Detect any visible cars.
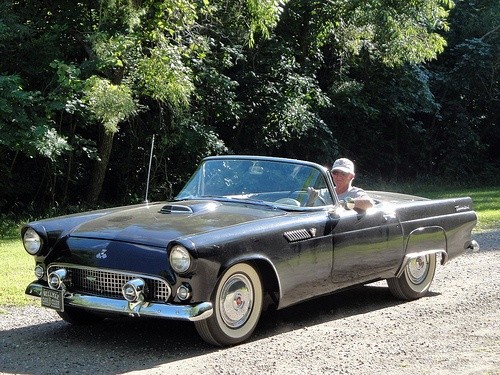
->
[22,156,480,348]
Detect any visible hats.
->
[330,157,354,174]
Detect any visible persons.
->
[302,158,376,209]
